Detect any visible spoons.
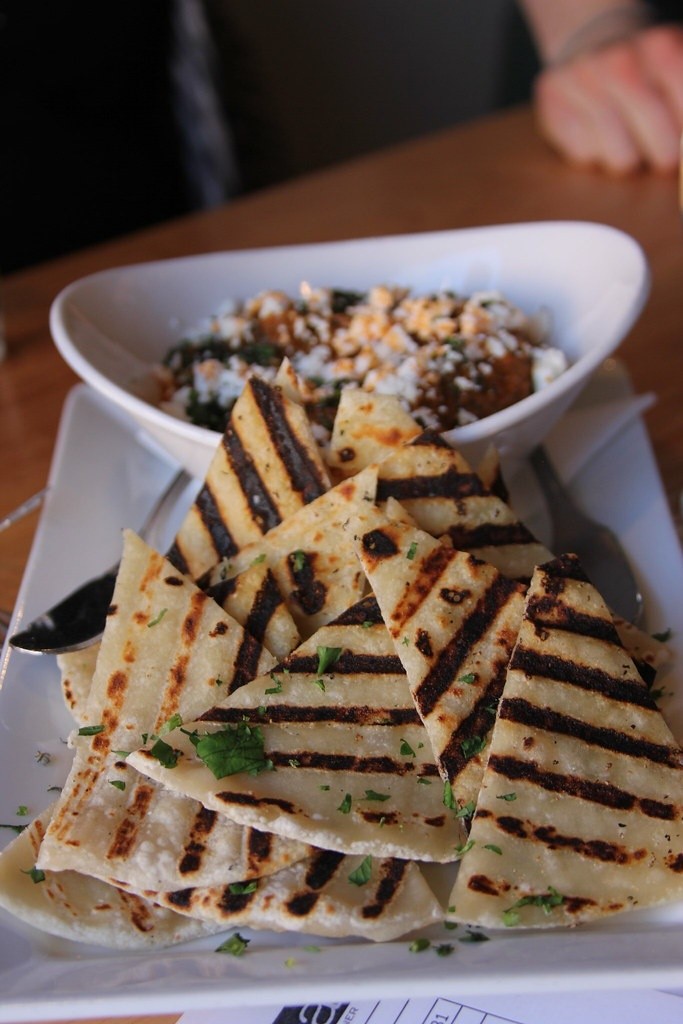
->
[5,468,196,658]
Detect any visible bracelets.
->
[550,8,651,66]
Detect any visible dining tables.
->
[0,97,683,1024]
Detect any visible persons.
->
[1,0,683,276]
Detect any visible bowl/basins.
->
[49,221,653,486]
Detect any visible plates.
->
[0,359,683,1022]
